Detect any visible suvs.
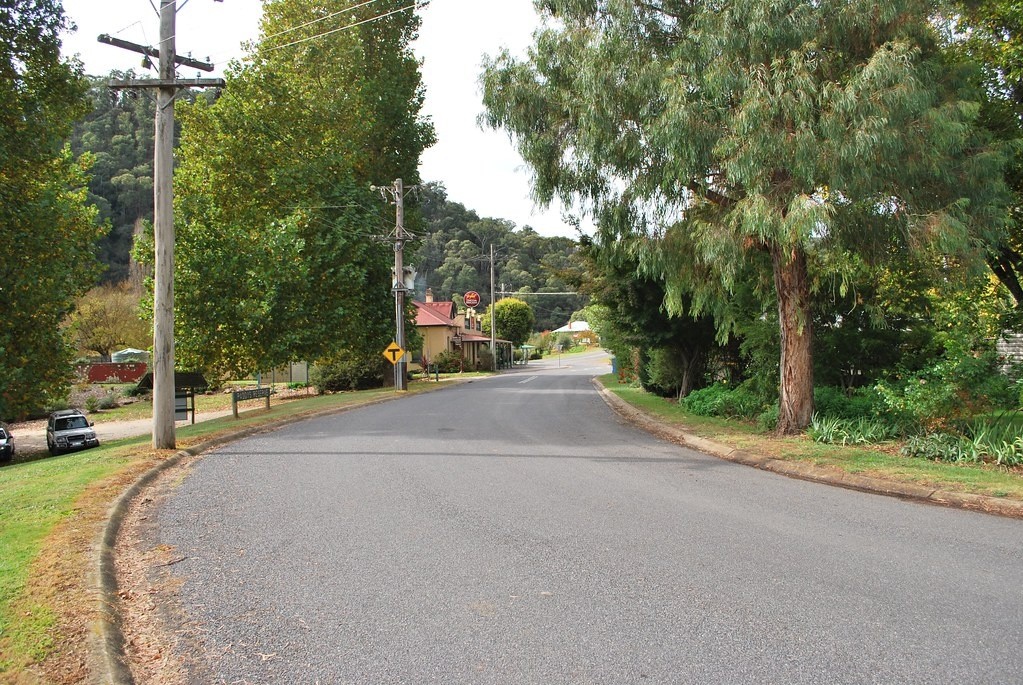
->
[47,408,99,455]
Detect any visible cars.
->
[0,427,15,463]
